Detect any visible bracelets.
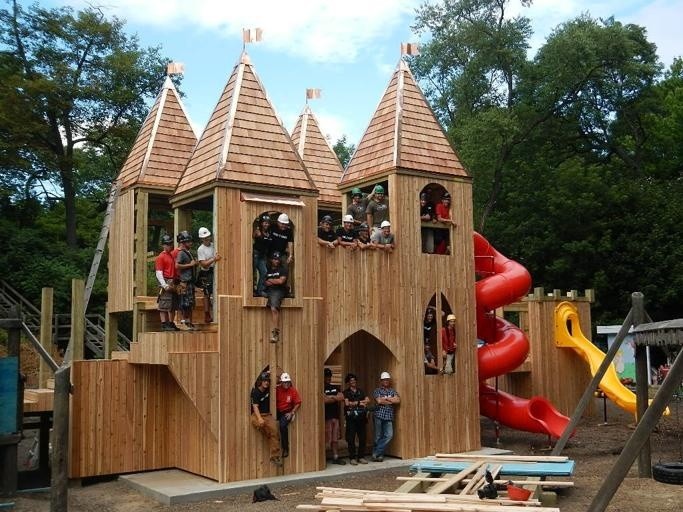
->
[211,256,216,262]
[385,396,388,401]
[356,400,360,406]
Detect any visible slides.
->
[474,230,576,439]
[554,302,670,418]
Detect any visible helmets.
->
[259,372,292,382]
[375,185,384,193]
[440,192,450,199]
[447,314,456,321]
[345,374,355,383]
[261,213,290,224]
[381,372,391,379]
[273,252,280,259]
[420,193,428,200]
[351,188,361,197]
[162,227,211,243]
[324,368,332,377]
[322,215,390,231]
[426,308,435,314]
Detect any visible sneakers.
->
[333,453,382,465]
[161,321,199,331]
[271,449,288,466]
[271,329,279,342]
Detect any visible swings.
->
[653,341,683,484]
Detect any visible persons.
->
[421,303,458,375]
[253,213,295,298]
[175,230,197,332]
[322,366,346,465]
[369,370,401,462]
[340,372,371,466]
[261,250,289,343]
[196,226,222,324]
[158,232,184,325]
[316,183,451,254]
[252,216,271,297]
[275,371,303,458]
[249,370,283,466]
[154,234,179,332]
